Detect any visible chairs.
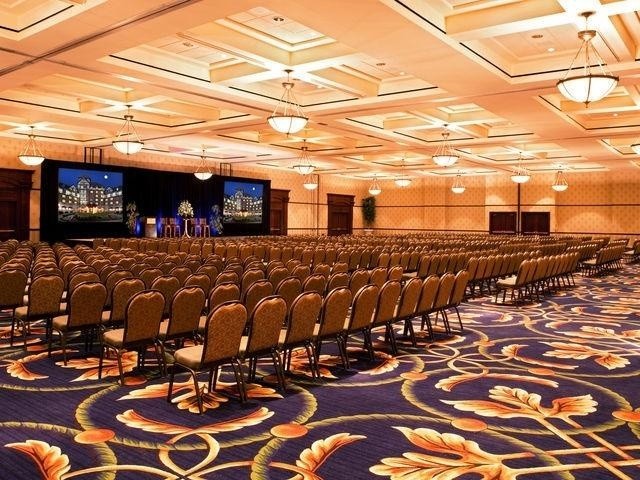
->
[159,218,181,238]
[190,218,211,239]
